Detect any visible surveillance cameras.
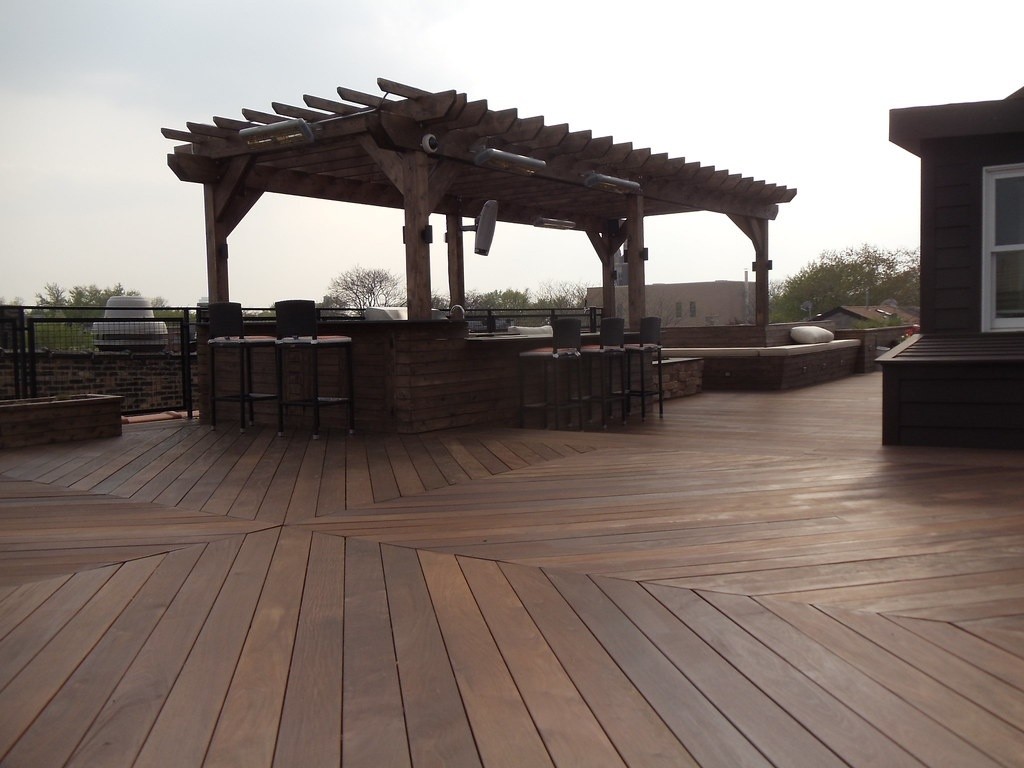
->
[423,133,439,154]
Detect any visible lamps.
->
[467,135,546,176]
[533,217,575,229]
[239,118,314,152]
[584,171,640,195]
[460,199,498,255]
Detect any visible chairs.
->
[517,318,663,433]
[207,300,354,439]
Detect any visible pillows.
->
[790,325,834,344]
